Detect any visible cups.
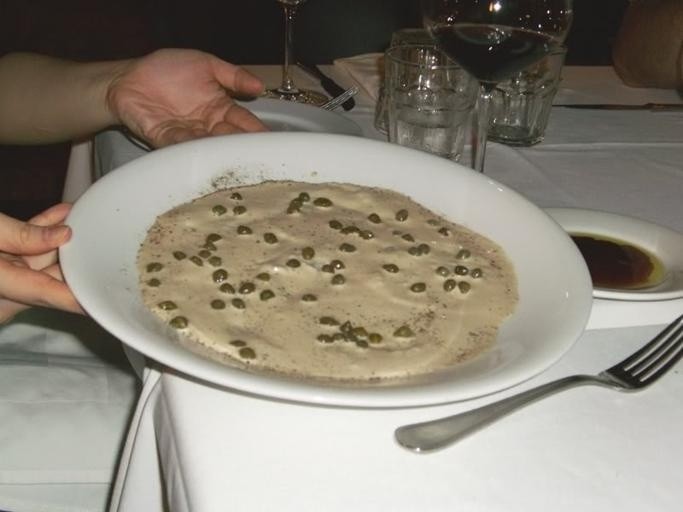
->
[384,45,476,162]
[485,46,565,147]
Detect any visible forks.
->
[394,314,681,453]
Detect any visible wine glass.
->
[419,1,574,178]
[262,0,329,106]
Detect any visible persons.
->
[0,46,273,329]
[612,0,683,90]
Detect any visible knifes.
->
[298,59,356,111]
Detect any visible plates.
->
[233,97,364,138]
[537,204,683,302]
[56,132,594,411]
